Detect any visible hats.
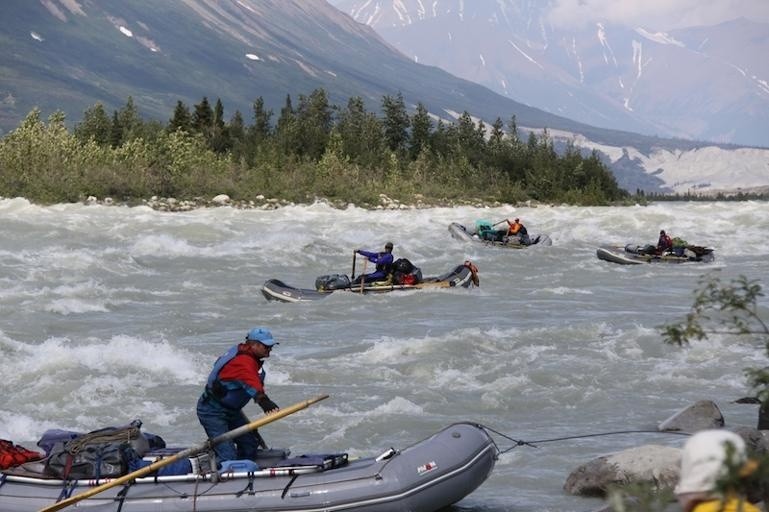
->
[385,243,393,249]
[673,430,758,493]
[246,328,280,347]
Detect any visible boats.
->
[260,264,473,303]
[449,222,551,252]
[597,243,714,264]
[0,422,497,511]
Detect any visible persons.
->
[196,326,281,469]
[507,217,523,235]
[655,230,672,255]
[354,241,394,284]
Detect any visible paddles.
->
[37,393,330,512]
[504,223,513,246]
